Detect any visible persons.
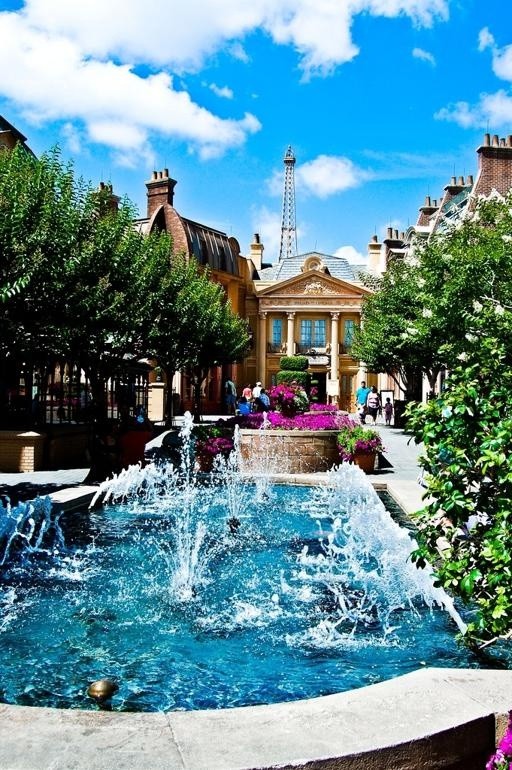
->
[382,397,393,426]
[222,374,322,416]
[366,385,383,426]
[353,380,371,425]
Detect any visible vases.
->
[353,451,376,474]
[194,452,214,473]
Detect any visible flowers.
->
[190,425,233,456]
[335,425,387,465]
[238,379,361,431]
